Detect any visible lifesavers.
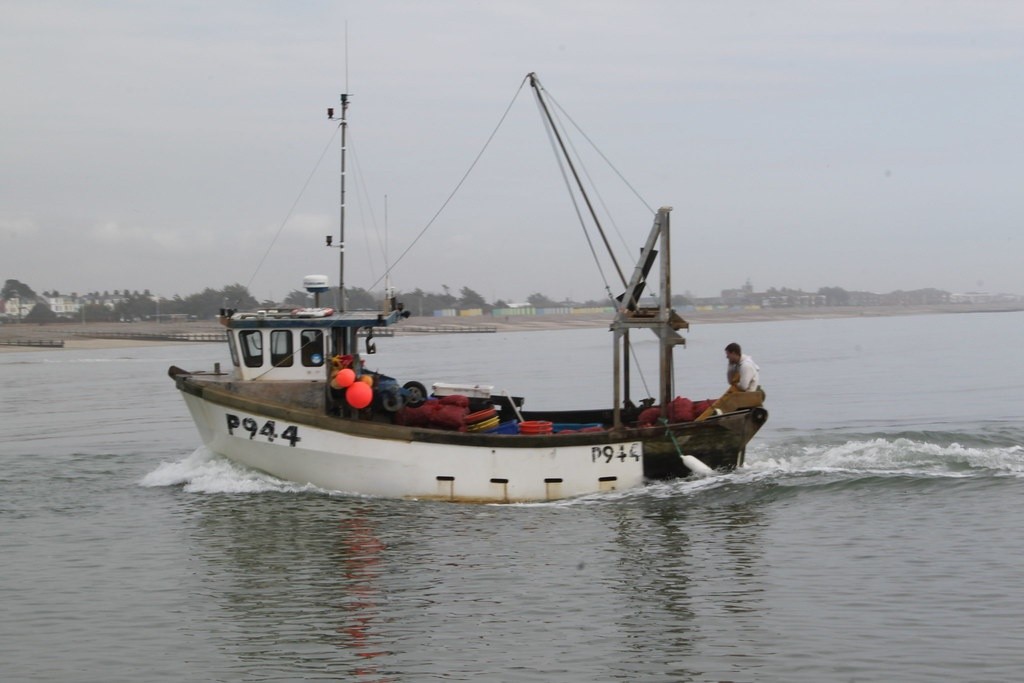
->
[518,420,554,434]
[464,407,500,433]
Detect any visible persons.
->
[724,343,760,392]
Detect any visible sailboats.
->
[168,16,768,503]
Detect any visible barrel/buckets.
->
[463,408,554,434]
